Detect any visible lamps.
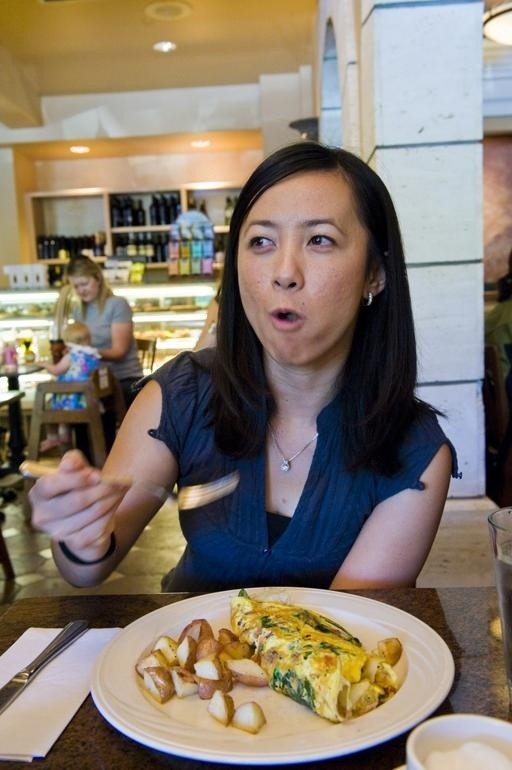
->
[482,0,512,49]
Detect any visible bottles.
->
[38,192,238,285]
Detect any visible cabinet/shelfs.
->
[0,281,220,375]
[24,179,250,271]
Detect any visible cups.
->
[50,340,64,365]
[488,505,511,698]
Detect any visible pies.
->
[229,595,395,722]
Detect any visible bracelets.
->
[56,531,117,566]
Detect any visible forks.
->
[19,461,241,511]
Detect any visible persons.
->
[25,141,462,591]
[34,322,103,455]
[59,255,145,412]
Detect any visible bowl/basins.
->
[407,713,512,767]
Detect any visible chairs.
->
[22,366,126,523]
[484,344,512,475]
[133,337,156,376]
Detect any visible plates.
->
[89,584,457,765]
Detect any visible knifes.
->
[0,618,90,713]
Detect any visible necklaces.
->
[268,420,319,472]
